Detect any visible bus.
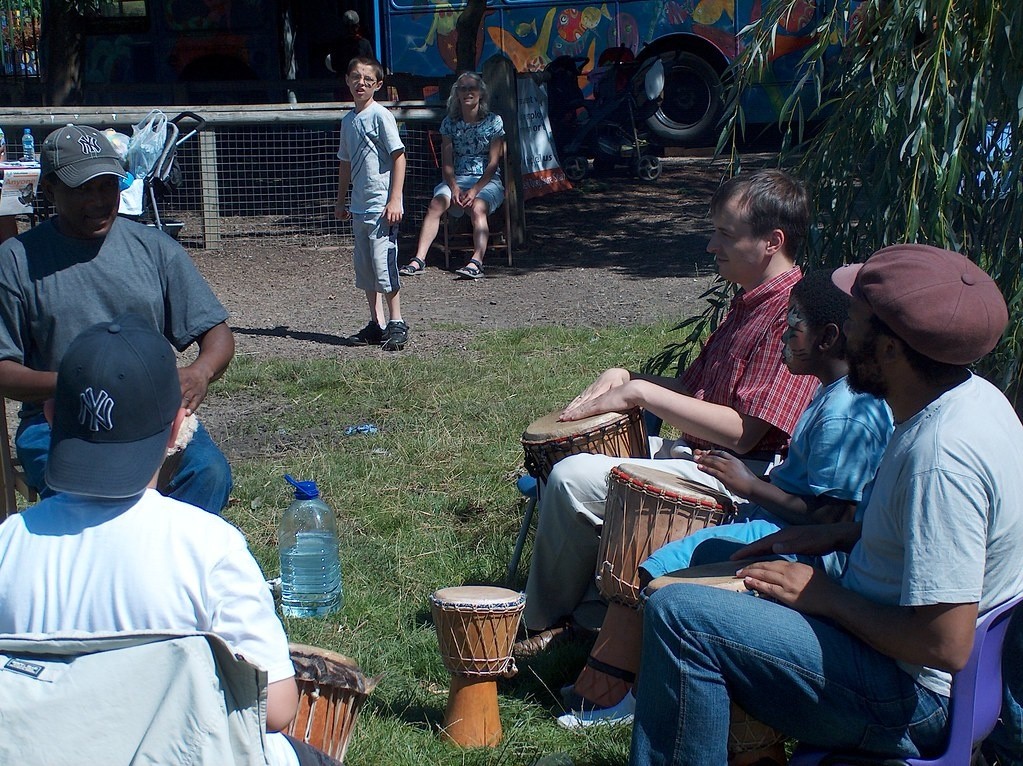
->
[374,0,909,148]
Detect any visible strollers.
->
[114,109,207,239]
[543,41,682,184]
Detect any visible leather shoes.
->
[513,618,602,661]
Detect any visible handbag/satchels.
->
[153,151,183,194]
[127,108,167,178]
[644,59,665,100]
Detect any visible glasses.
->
[455,85,481,93]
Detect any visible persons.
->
[400,71,505,278]
[331,10,373,73]
[522,165,1023,766]
[0,125,236,516]
[0,317,344,766]
[334,57,409,350]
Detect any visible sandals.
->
[398,257,426,275]
[455,258,485,279]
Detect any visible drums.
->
[522,404,652,486]
[571,463,737,713]
[428,585,527,748]
[278,644,363,764]
[641,552,787,765]
[156,411,199,492]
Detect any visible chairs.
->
[442,137,524,266]
[788,595,1023,766]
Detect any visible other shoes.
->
[557,687,640,730]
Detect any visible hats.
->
[43,313,179,499]
[343,9,359,25]
[39,124,128,188]
[831,242,1007,367]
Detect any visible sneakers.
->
[349,320,389,344]
[388,321,409,347]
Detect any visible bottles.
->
[279,473,343,619]
[22,128,35,162]
